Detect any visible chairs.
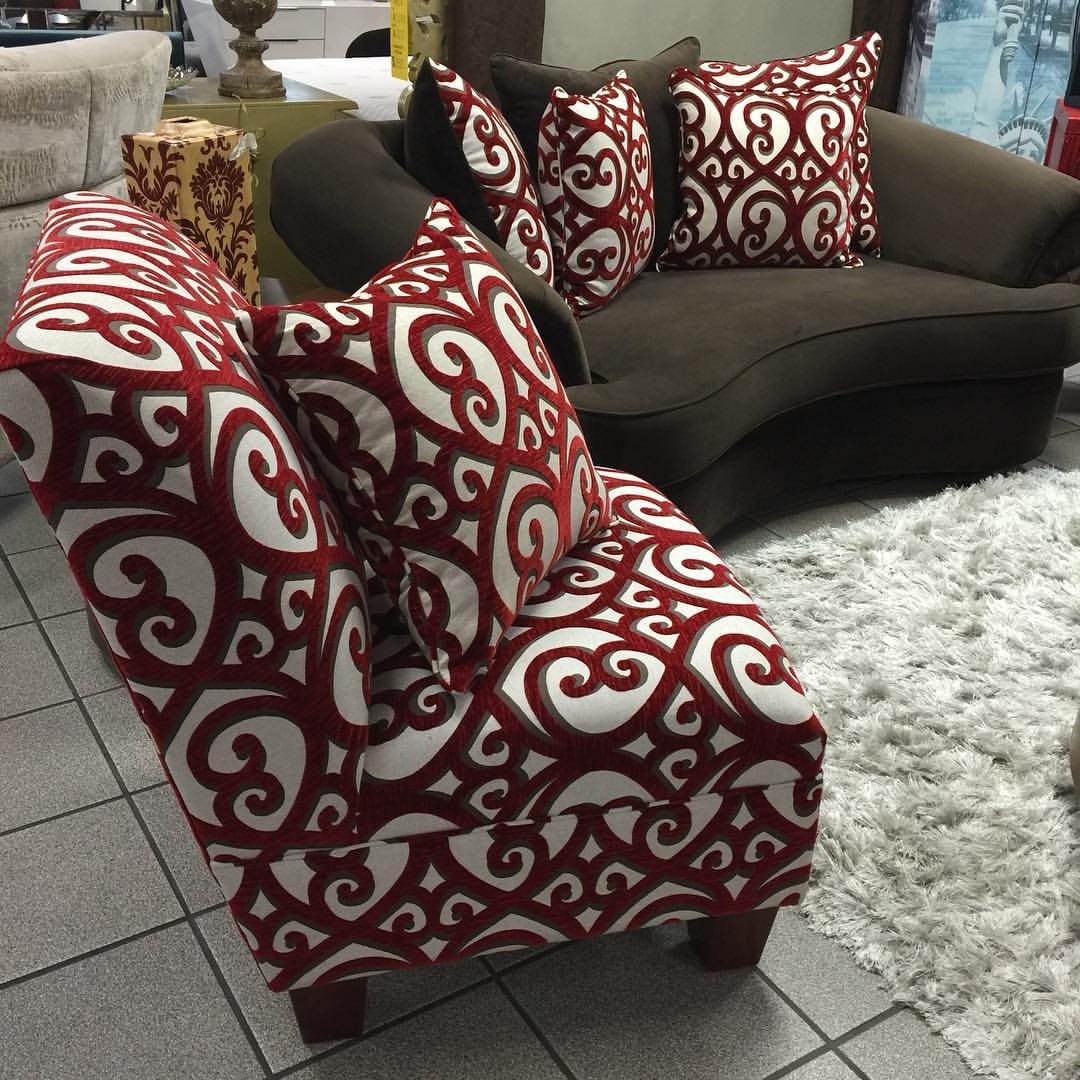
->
[0,29,172,330]
[0,192,823,1044]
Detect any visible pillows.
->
[238,34,880,692]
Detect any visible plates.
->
[166,63,200,91]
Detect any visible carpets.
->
[718,463,1080,1080]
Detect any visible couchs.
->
[267,105,1080,543]
